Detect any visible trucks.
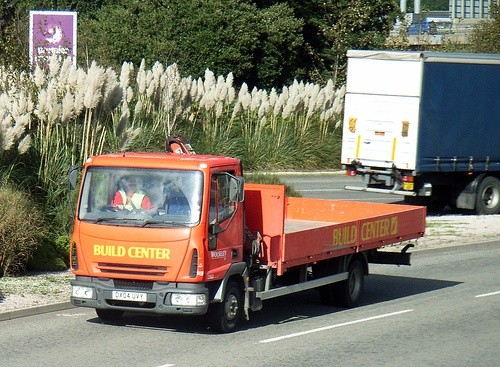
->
[340,49,500,215]
[68,151,428,333]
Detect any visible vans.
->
[405,21,437,36]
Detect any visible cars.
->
[429,21,454,35]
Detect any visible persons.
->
[110,176,155,212]
